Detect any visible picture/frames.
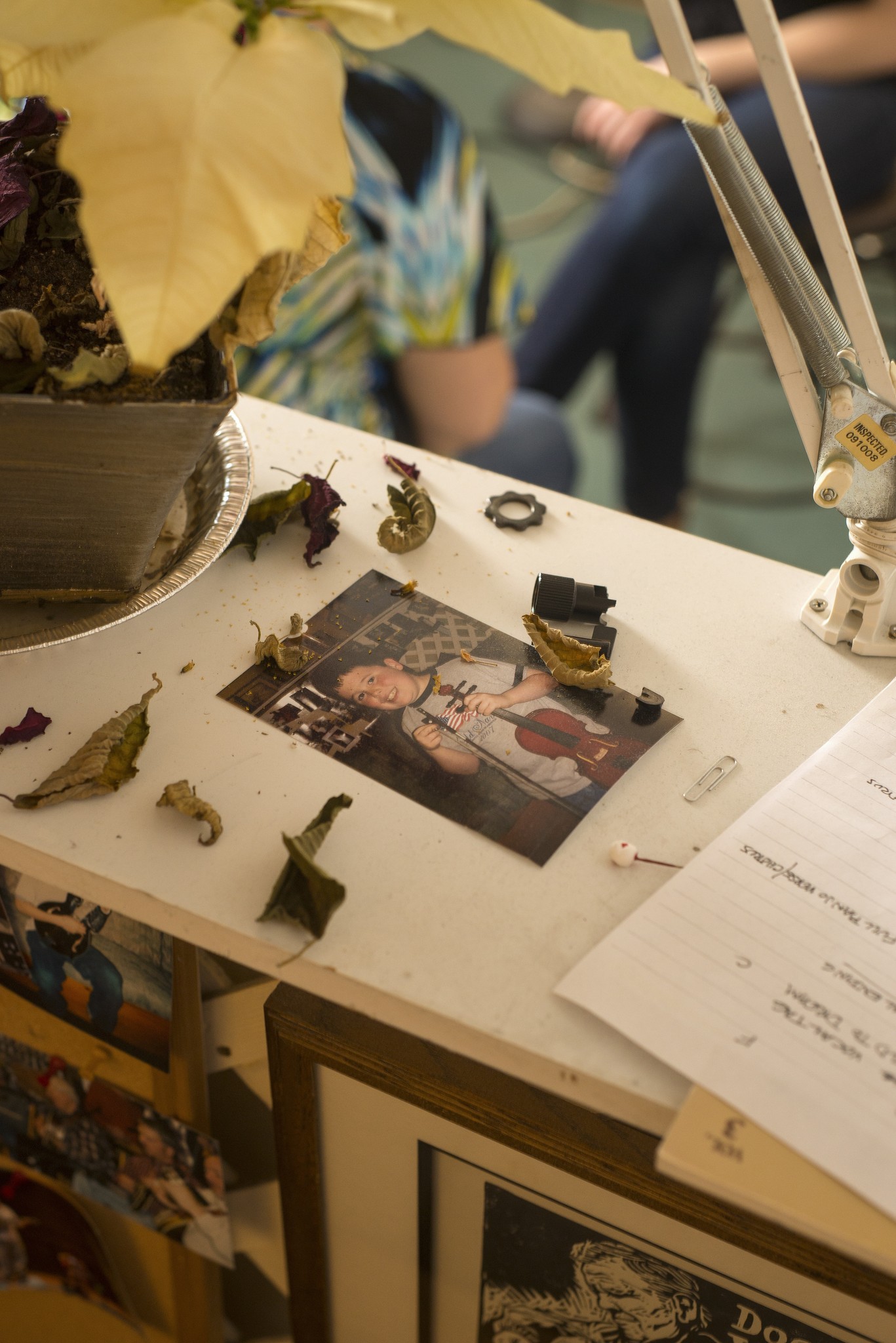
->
[263,985,896,1343]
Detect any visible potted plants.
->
[0,1,434,607]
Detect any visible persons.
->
[306,645,631,820]
[15,1057,237,1270]
[208,0,896,538]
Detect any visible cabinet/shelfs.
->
[3,393,896,1343]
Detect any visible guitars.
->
[34,892,113,959]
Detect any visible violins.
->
[437,681,652,788]
